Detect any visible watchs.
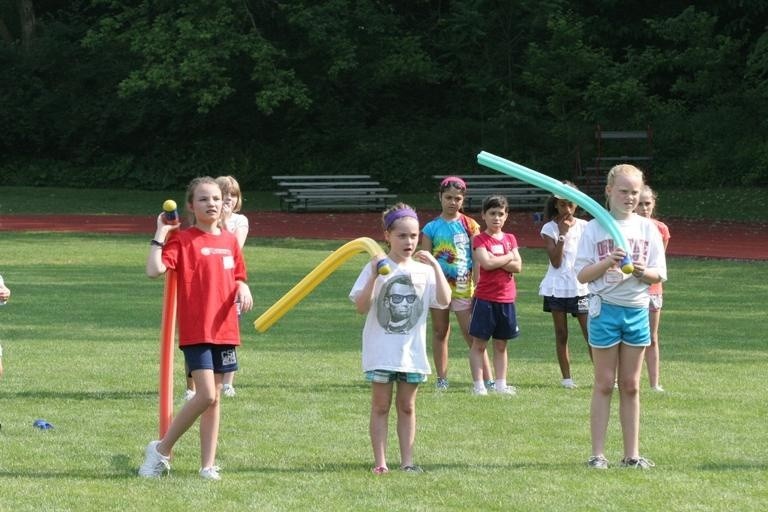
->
[558,234,566,242]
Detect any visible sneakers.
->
[199,466,221,480]
[436,379,447,392]
[185,391,195,400]
[587,455,608,469]
[139,442,170,477]
[473,380,516,394]
[620,457,655,469]
[224,386,234,399]
[561,381,575,388]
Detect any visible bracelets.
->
[150,240,164,247]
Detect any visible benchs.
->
[432,170,554,214]
[273,174,400,214]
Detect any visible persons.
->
[138,175,253,477]
[538,179,621,390]
[184,176,250,397]
[575,164,668,467]
[633,184,671,391]
[0,269,11,376]
[420,176,495,390]
[467,193,521,395]
[349,202,452,474]
